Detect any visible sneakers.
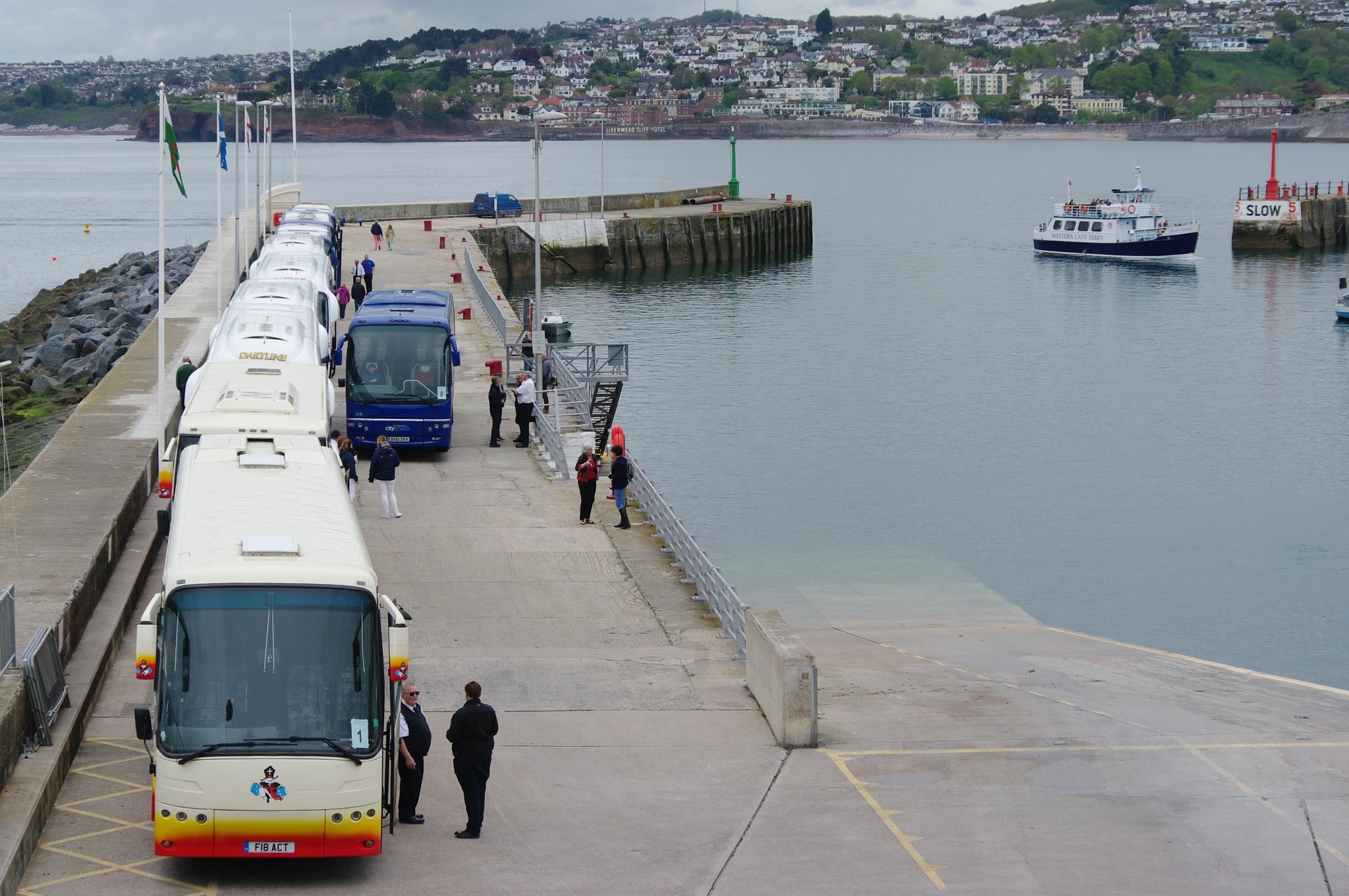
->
[513,436,520,439]
[399,813,424,824]
[543,409,549,414]
[395,513,402,517]
[454,831,480,839]
[489,443,500,447]
[415,814,424,819]
[381,513,389,518]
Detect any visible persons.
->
[541,353,551,414]
[351,276,367,314]
[486,368,537,453]
[339,438,359,502]
[384,225,394,252]
[521,330,535,372]
[368,436,403,519]
[608,445,632,530]
[360,254,376,296]
[360,364,381,385]
[350,260,365,284]
[1064,197,1111,219]
[329,429,344,468]
[444,680,499,840]
[575,443,602,526]
[370,220,383,251]
[396,682,432,825]
[175,355,198,412]
[335,282,351,320]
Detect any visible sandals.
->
[580,520,585,524]
[585,519,596,524]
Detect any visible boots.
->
[614,507,626,527]
[619,508,631,529]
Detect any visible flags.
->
[217,110,230,172]
[163,94,188,199]
[244,108,252,153]
[264,108,271,144]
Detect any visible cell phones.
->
[525,335,529,338]
[510,389,513,391]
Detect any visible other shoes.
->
[390,248,392,251]
[341,318,344,320]
[374,248,377,250]
[378,246,380,249]
[388,248,390,250]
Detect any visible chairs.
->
[355,333,446,385]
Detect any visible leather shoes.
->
[513,437,520,442]
[498,436,505,440]
[515,444,528,448]
[496,437,501,441]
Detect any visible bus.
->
[170,357,336,451]
[132,428,415,863]
[209,202,345,369]
[340,288,460,454]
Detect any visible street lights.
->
[594,112,605,219]
[232,99,285,296]
[530,111,570,409]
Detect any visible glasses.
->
[403,691,419,696]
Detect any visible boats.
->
[541,308,573,343]
[1028,164,1201,260]
[1333,277,1349,320]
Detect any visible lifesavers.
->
[1080,205,1086,214]
[1128,205,1136,214]
[1064,204,1070,214]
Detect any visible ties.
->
[355,265,358,276]
